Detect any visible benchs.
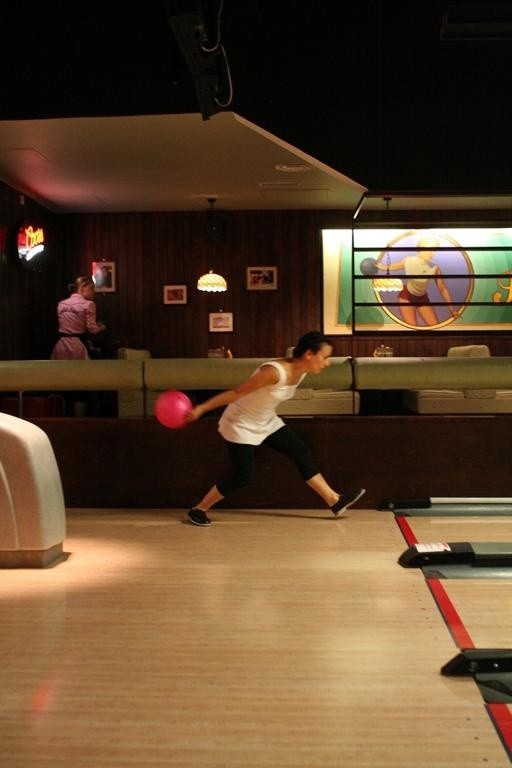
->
[415,345,512,413]
[118,348,162,419]
[276,347,360,415]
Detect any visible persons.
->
[51,277,107,417]
[184,332,366,526]
[377,237,463,328]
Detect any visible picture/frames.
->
[91,260,117,294]
[245,266,279,291]
[162,284,189,306]
[197,198,227,292]
[208,312,235,334]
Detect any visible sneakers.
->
[331,488,366,518]
[188,509,211,526]
